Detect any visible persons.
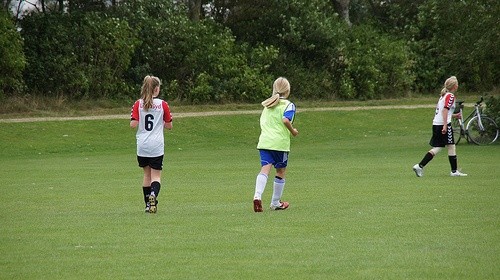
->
[413,76,466,178]
[253,76,298,214]
[131,76,173,213]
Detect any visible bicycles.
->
[451,96,499,145]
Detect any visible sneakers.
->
[253,200,263,212]
[411,163,424,177]
[149,194,158,214]
[144,202,149,211]
[450,170,468,177]
[269,201,289,210]
